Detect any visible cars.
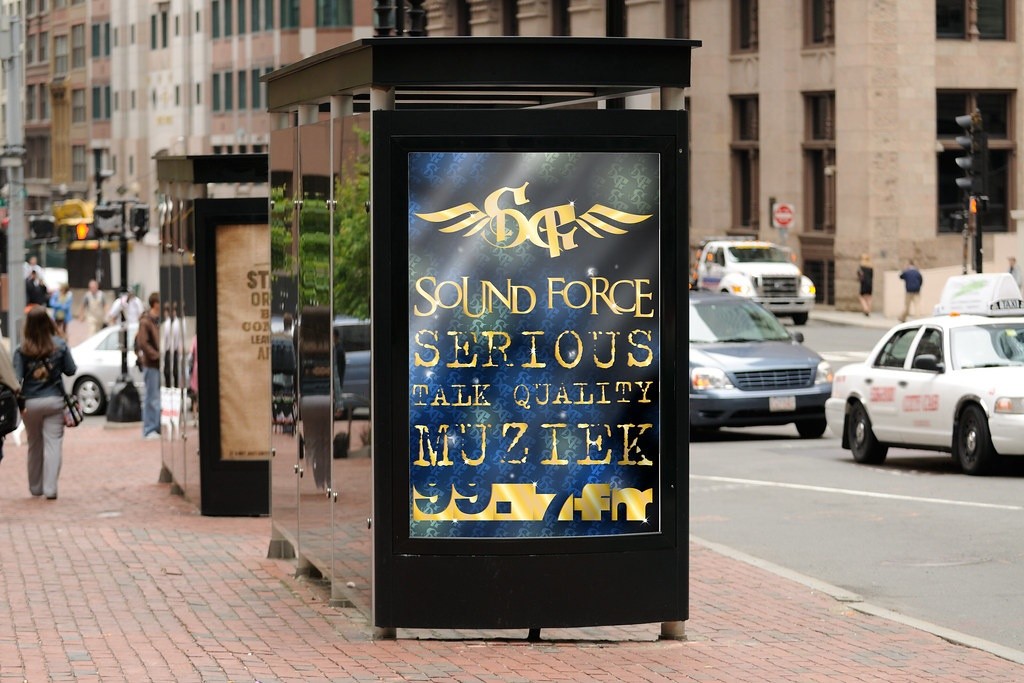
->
[691,291,836,439]
[332,319,371,414]
[63,322,141,418]
[825,272,1024,478]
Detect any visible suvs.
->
[698,241,817,325]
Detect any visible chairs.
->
[920,342,942,363]
[750,250,764,259]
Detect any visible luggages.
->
[333,405,353,459]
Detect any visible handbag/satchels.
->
[63,395,84,428]
[106,376,142,422]
[0,383,20,438]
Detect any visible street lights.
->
[94,198,151,424]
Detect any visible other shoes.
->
[147,430,161,439]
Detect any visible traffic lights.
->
[953,106,980,197]
[56,217,97,249]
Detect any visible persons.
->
[138,292,161,438]
[898,264,922,323]
[26,256,72,333]
[857,257,873,316]
[12,313,76,500]
[1008,258,1022,289]
[160,303,198,427]
[80,281,145,334]
[0,339,26,414]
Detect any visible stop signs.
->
[771,203,794,231]
